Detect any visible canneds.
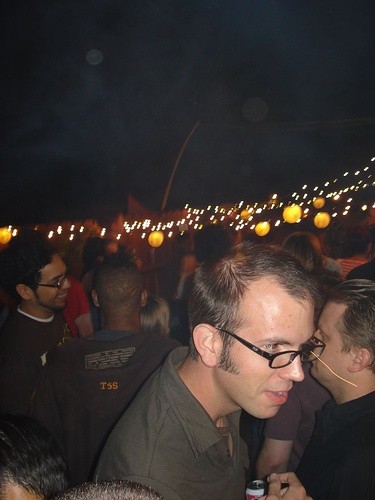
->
[245,479,269,500]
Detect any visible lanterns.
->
[313,197,325,208]
[255,221,270,237]
[314,212,330,229]
[241,210,250,220]
[0,226,11,244]
[148,231,164,247]
[282,205,302,223]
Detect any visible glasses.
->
[216,324,329,368]
[38,279,67,293]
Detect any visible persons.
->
[0,230,70,417]
[30,257,170,488]
[0,207,375,352]
[0,414,70,500]
[255,275,334,481]
[51,480,167,500]
[94,239,311,500]
[295,279,375,500]
